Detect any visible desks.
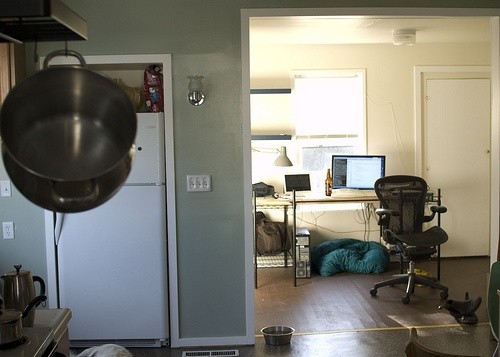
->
[252,187,435,290]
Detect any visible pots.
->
[1,48,139,215]
[0,295,47,347]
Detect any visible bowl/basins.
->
[261,325,295,345]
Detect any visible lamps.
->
[251,144,294,167]
[185,75,204,106]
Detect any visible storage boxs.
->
[262,325,296,346]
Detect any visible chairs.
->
[374,174,449,305]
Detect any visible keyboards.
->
[331,189,379,198]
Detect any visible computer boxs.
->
[294,227,310,278]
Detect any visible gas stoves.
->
[0,306,73,357]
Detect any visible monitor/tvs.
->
[331,154,386,190]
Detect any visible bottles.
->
[325,168,333,197]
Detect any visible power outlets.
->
[0,180,13,198]
[3,221,15,241]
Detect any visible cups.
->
[0,264,46,327]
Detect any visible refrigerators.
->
[55,111,170,348]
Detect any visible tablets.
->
[284,174,311,192]
